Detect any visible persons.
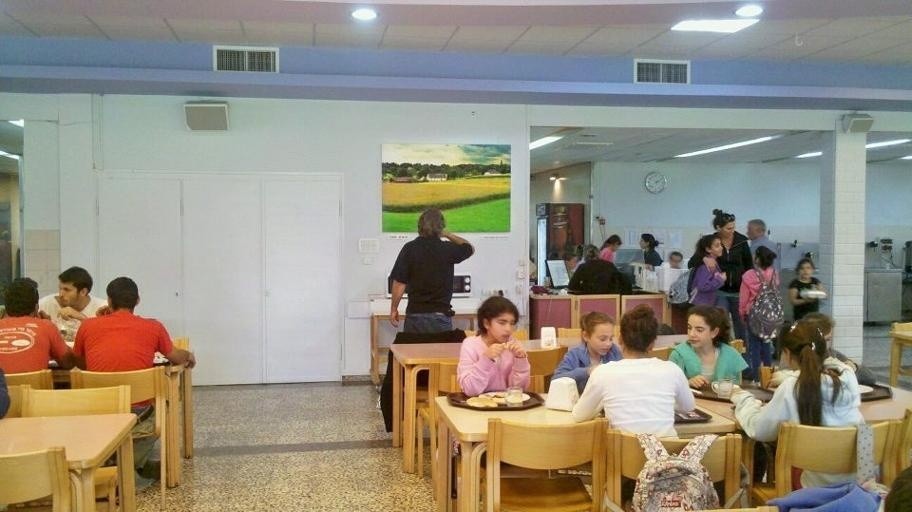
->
[549,311,625,394]
[600,234,623,264]
[687,209,756,380]
[71,277,197,494]
[689,235,727,306]
[388,209,474,334]
[571,303,696,499]
[639,234,664,266]
[789,258,827,319]
[457,296,531,397]
[747,218,780,272]
[661,252,687,293]
[668,304,749,389]
[738,246,785,381]
[803,311,876,385]
[713,319,873,489]
[0,277,75,373]
[38,267,108,348]
[568,244,633,295]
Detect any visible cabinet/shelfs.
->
[371,310,478,395]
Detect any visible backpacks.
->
[667,261,704,305]
[747,268,785,344]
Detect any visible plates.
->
[478,390,530,405]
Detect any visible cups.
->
[507,386,524,409]
[711,380,733,399]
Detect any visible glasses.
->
[716,213,737,227]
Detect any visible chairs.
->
[486,418,609,512]
[622,293,668,332]
[578,293,620,331]
[2,447,76,512]
[747,420,903,512]
[529,295,572,340]
[20,386,132,512]
[70,367,171,507]
